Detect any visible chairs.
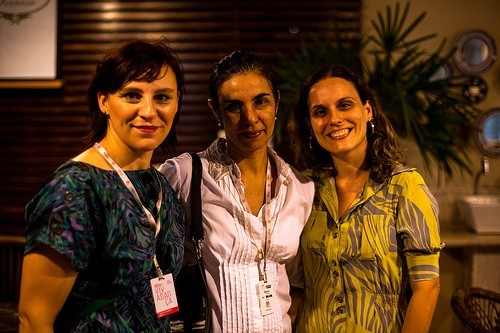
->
[450,286,500,333]
[0,235,27,333]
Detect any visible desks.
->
[435,219,500,333]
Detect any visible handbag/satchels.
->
[163,152,210,333]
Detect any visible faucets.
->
[473,156,490,196]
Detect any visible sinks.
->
[455,195,500,235]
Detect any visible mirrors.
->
[455,29,496,73]
[474,106,500,158]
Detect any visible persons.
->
[157,49,315,333]
[17,35,185,332]
[290,61,445,333]
